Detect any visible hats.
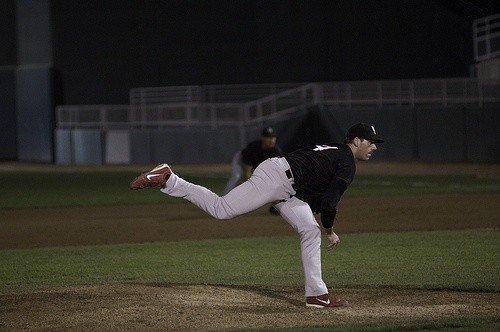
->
[261,128,277,138]
[347,123,385,143]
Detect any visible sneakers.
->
[131,163,173,189]
[305,292,348,307]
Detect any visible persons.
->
[129,122,385,308]
[223,125,286,216]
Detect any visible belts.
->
[277,155,297,193]
